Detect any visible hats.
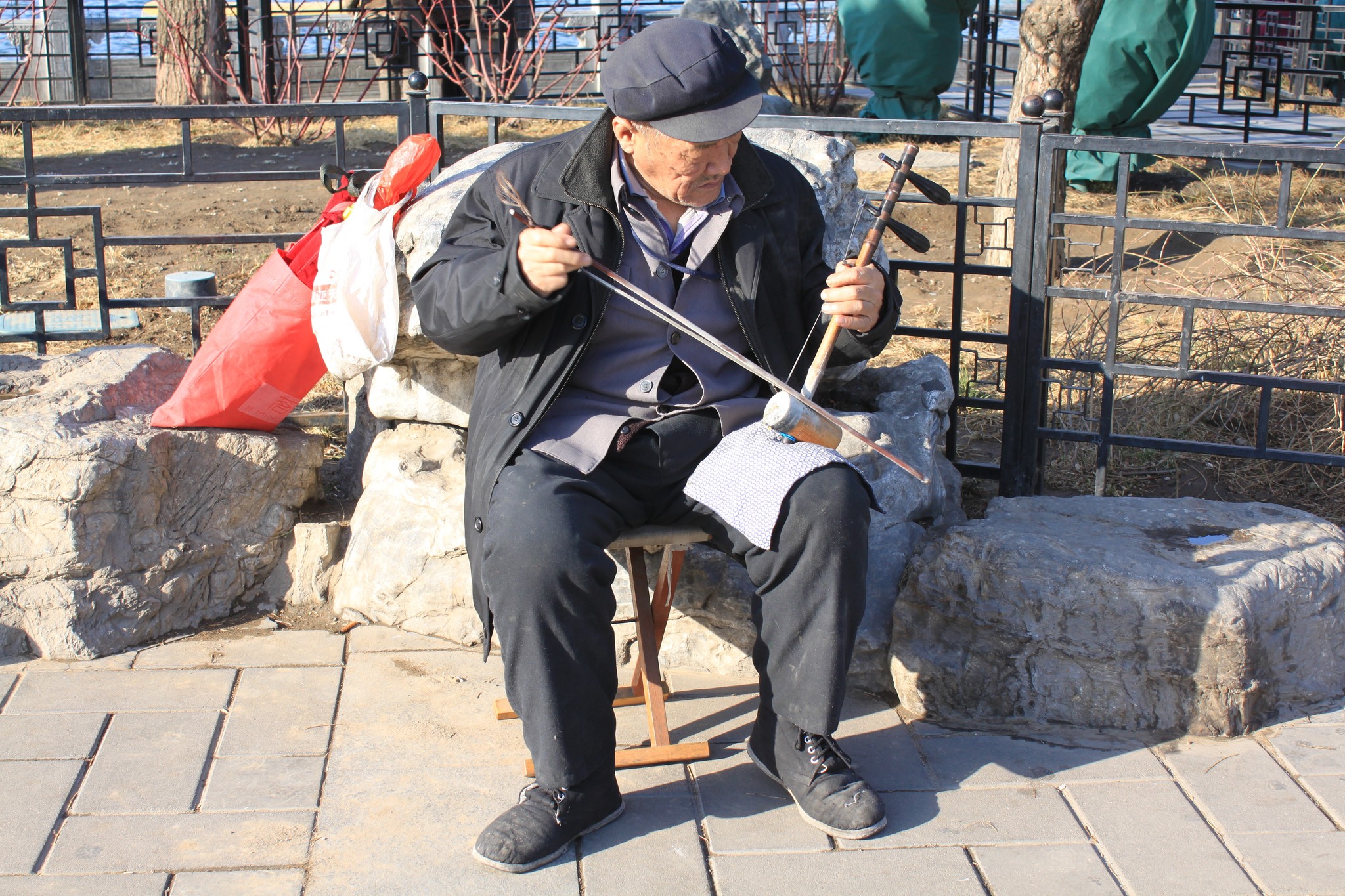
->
[600,17,763,143]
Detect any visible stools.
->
[495,526,713,777]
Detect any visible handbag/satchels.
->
[311,172,412,380]
[151,201,356,432]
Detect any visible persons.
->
[411,18,902,873]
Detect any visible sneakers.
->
[746,702,887,840]
[475,780,626,873]
